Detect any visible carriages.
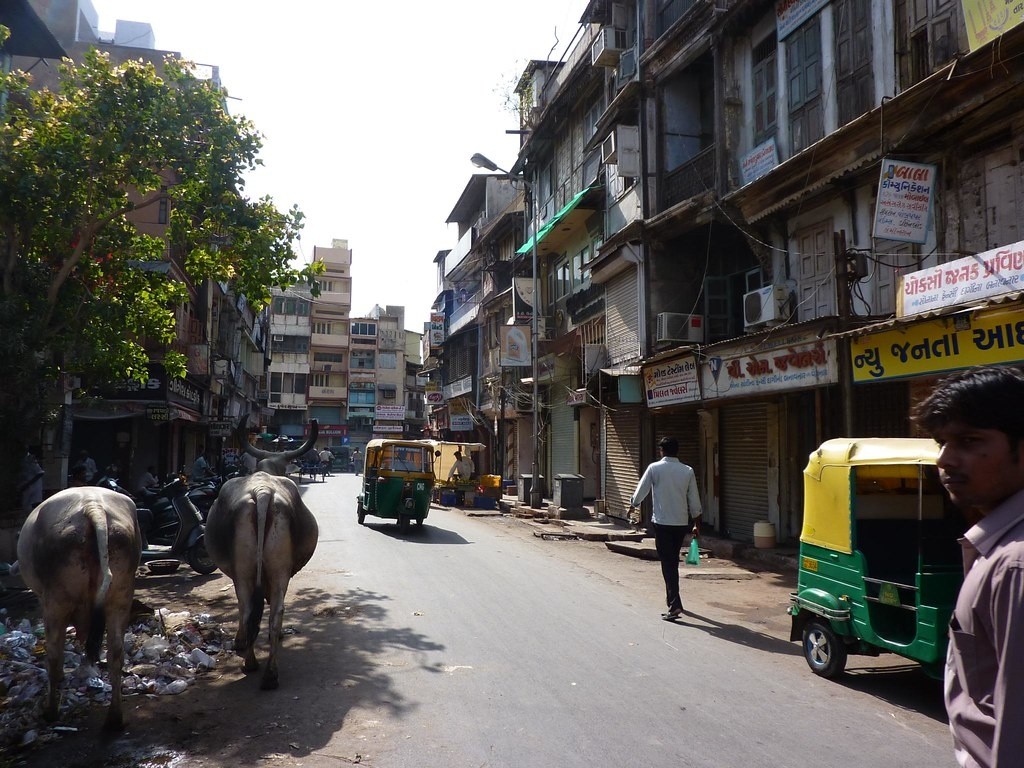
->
[296,448,326,484]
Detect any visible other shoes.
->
[662,605,681,620]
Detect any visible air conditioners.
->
[744,284,790,327]
[274,335,284,341]
[601,131,617,164]
[657,312,704,342]
[515,393,543,412]
[384,390,395,398]
[591,29,632,68]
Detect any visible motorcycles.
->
[357,438,441,536]
[789,438,970,681]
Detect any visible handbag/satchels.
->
[685,532,698,565]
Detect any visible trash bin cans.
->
[518,473,544,502]
[553,474,587,508]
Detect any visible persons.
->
[446,451,475,485]
[76,449,98,481]
[277,442,287,452]
[199,445,205,456]
[391,449,415,471]
[144,467,158,488]
[193,448,216,477]
[305,448,319,463]
[319,447,335,464]
[15,446,45,517]
[353,447,362,476]
[240,452,256,468]
[627,437,702,619]
[912,365,1024,768]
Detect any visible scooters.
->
[99,464,243,576]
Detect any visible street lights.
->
[472,153,542,510]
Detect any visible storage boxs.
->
[442,475,517,509]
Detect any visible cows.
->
[202,412,319,694]
[16,485,142,736]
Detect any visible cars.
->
[329,446,355,473]
